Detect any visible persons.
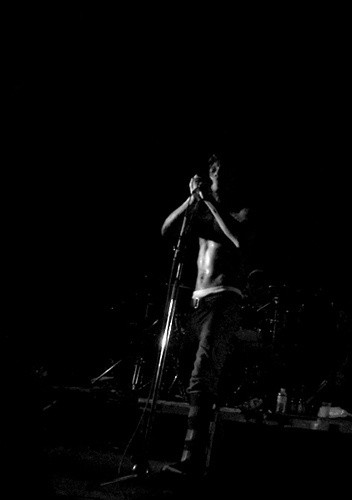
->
[120,151,248,485]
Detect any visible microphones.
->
[191,174,206,203]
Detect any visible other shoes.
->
[172,458,207,478]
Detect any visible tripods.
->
[101,188,200,484]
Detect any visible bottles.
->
[276,388,288,415]
[289,384,305,415]
